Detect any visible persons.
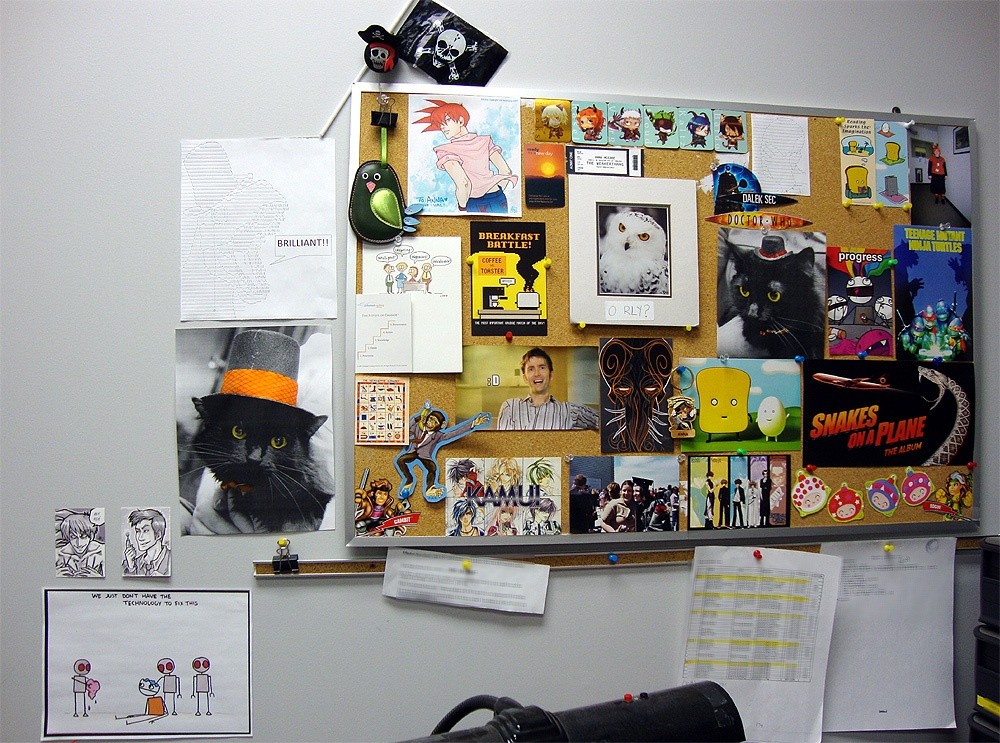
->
[497,348,599,431]
[569,472,679,532]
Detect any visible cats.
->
[722,237,826,356]
[190,330,334,532]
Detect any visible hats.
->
[632,476,654,491]
